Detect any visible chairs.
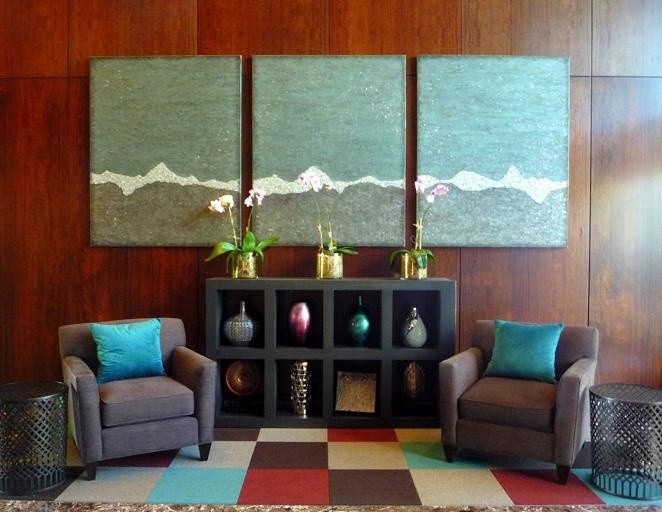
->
[58,317,217,480]
[438,320,600,484]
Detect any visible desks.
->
[589,383,662,500]
[0,380,68,496]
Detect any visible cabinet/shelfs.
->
[206,276,457,427]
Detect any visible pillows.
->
[87,317,167,385]
[483,320,565,384]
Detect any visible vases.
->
[231,252,257,278]
[317,252,343,279]
[401,253,428,280]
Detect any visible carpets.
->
[0,423,662,504]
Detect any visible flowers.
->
[385,175,450,268]
[298,167,359,256]
[204,188,281,274]
